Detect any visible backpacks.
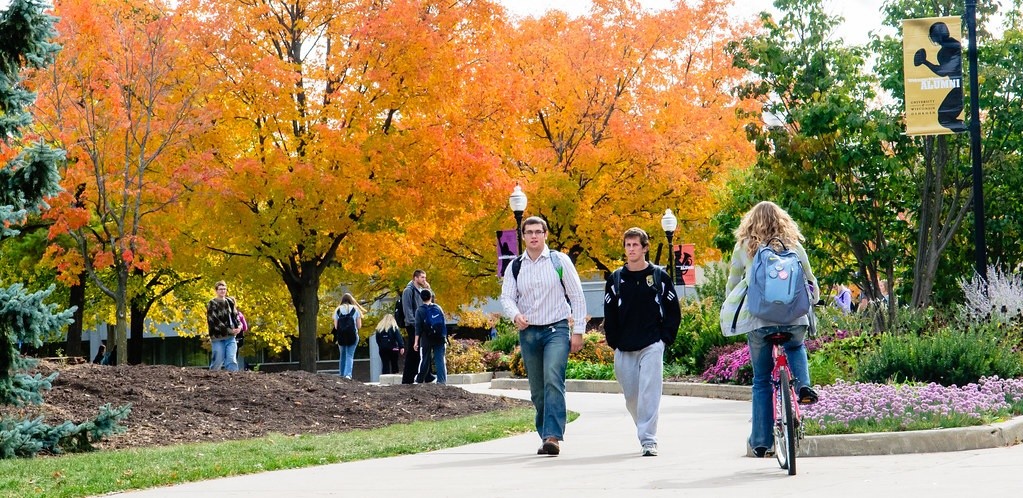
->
[747,236,811,324]
[379,328,394,352]
[394,285,416,328]
[420,304,445,338]
[335,306,356,347]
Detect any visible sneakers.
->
[641,443,658,456]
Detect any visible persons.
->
[93,346,118,365]
[604,227,681,456]
[501,217,587,455]
[719,200,819,457]
[207,281,247,371]
[830,272,896,332]
[375,270,448,384]
[332,293,367,380]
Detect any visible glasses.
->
[218,287,227,290]
[523,229,545,236]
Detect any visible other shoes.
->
[538,435,560,455]
[748,434,768,458]
[799,387,818,405]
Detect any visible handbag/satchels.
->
[842,292,856,312]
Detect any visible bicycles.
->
[758,332,813,476]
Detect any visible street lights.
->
[662,208,677,284]
[509,186,527,255]
